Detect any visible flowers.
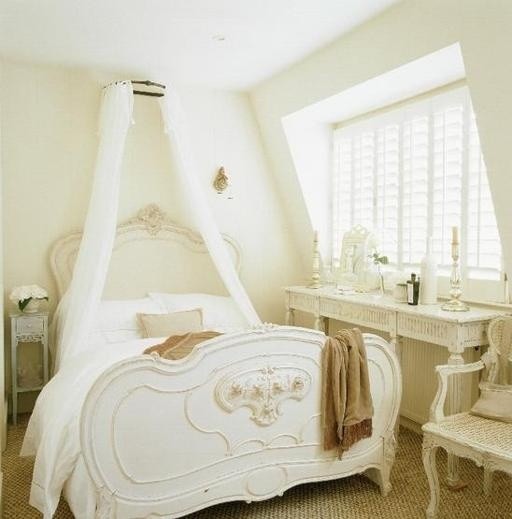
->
[369,235,390,293]
[10,285,50,313]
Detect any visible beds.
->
[46,204,403,519]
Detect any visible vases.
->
[19,299,42,312]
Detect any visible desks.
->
[283,286,498,492]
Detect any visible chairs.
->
[416,315,511,519]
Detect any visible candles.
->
[452,228,460,244]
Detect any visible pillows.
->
[474,378,512,423]
[99,293,246,344]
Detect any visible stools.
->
[8,314,53,424]
